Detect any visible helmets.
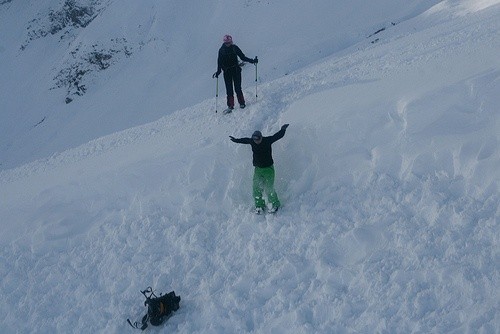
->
[224,35,232,41]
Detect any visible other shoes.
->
[228,106,233,109]
[240,104,245,108]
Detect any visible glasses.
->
[225,41,232,44]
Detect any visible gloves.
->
[229,136,236,142]
[212,73,219,78]
[281,124,289,131]
[254,59,258,63]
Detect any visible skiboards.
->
[223,103,246,113]
[255,205,279,214]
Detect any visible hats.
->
[252,131,262,139]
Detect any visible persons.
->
[213,35,258,111]
[229,123,289,215]
